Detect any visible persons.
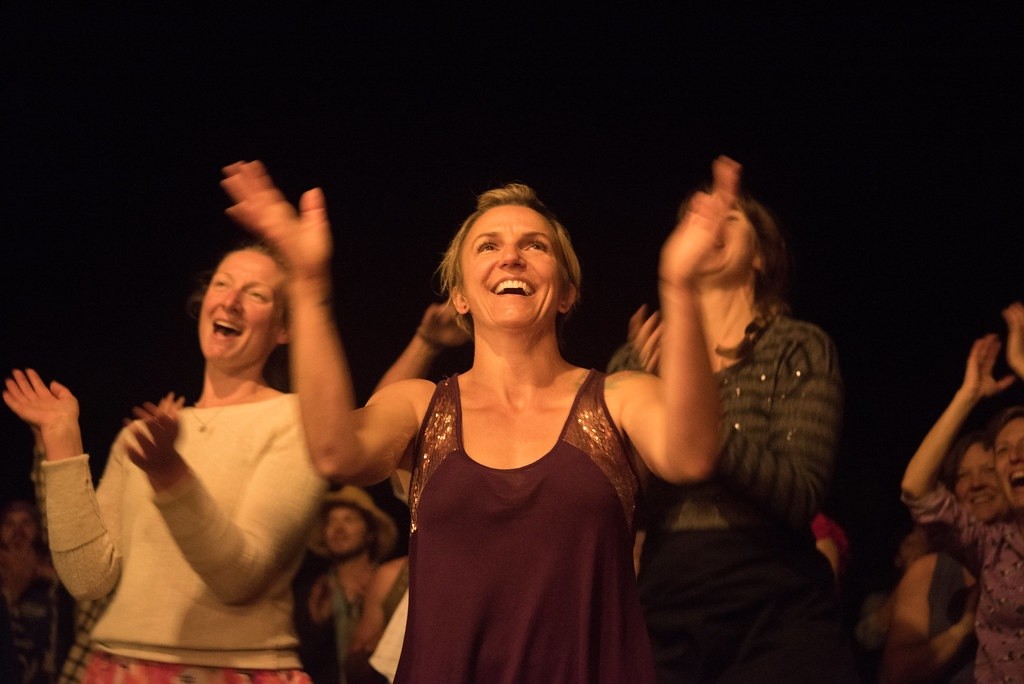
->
[0,498,79,684]
[222,157,744,684]
[607,190,847,683]
[297,480,413,684]
[3,245,331,684]
[811,301,1024,684]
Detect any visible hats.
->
[307,484,397,557]
[807,512,852,558]
